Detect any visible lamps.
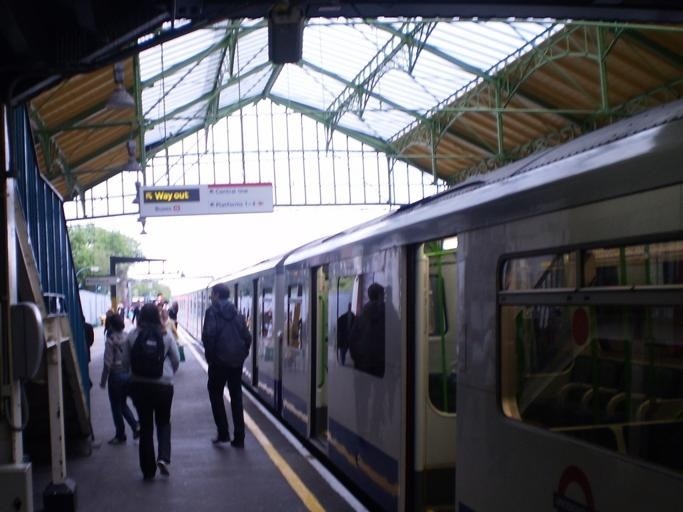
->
[105,60,136,109]
[122,124,141,171]
[131,171,141,204]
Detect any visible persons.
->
[101,292,184,364]
[351,282,401,447]
[125,303,180,482]
[200,284,252,448]
[335,303,358,367]
[98,314,146,444]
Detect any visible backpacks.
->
[214,318,246,368]
[130,322,164,379]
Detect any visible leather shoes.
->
[212,437,229,444]
[230,440,244,448]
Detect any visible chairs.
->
[528,354,681,453]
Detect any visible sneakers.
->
[145,473,155,480]
[133,420,141,439]
[107,435,126,444]
[157,460,169,479]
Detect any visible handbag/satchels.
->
[178,344,185,361]
